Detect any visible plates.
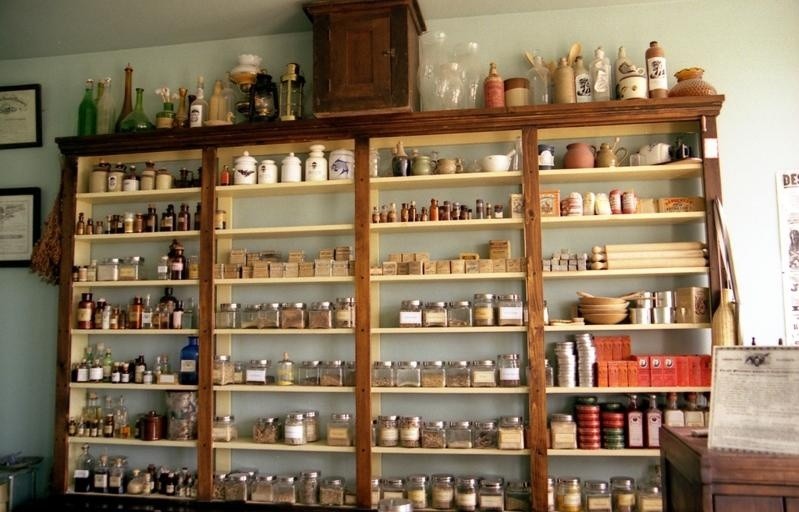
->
[578,294,630,323]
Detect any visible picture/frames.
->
[0,80,46,151]
[0,185,44,270]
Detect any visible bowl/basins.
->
[481,156,512,173]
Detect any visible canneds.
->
[372,353,553,387]
[399,294,549,327]
[375,392,710,450]
[214,410,356,446]
[97,257,142,280]
[377,464,661,512]
[214,467,357,505]
[213,354,355,388]
[215,296,355,328]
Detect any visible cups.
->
[539,144,555,170]
[630,152,646,166]
[597,143,628,167]
[390,141,465,175]
[565,143,597,169]
[631,287,709,323]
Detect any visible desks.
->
[0,455,46,511]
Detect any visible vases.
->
[115,64,136,131]
[117,86,158,132]
[667,64,717,98]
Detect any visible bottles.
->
[640,144,690,164]
[370,292,532,509]
[73,335,200,385]
[671,66,716,97]
[70,442,199,497]
[545,358,554,386]
[372,198,504,224]
[417,29,483,110]
[68,390,199,441]
[547,475,663,512]
[77,286,196,330]
[72,200,202,235]
[87,160,200,192]
[73,240,199,281]
[550,413,578,449]
[78,60,234,137]
[629,394,706,448]
[214,299,357,503]
[482,38,669,108]
[219,143,355,186]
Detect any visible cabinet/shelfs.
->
[658,424,799,511]
[303,0,429,117]
[53,92,727,512]
